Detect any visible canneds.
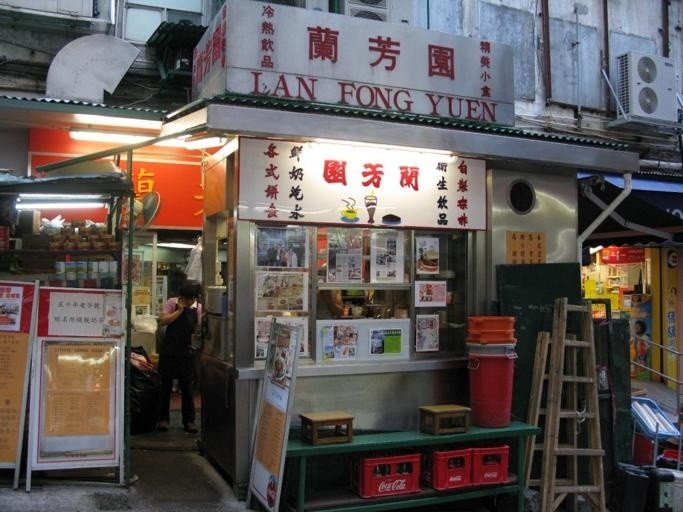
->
[55,261,119,281]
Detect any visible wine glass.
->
[365,196,377,223]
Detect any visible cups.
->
[341,209,358,219]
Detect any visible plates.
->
[340,217,358,223]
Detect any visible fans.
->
[126,191,161,231]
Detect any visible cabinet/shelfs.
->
[0,178,133,290]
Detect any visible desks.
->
[287,420,542,512]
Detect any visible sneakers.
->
[184,422,198,433]
[157,421,170,429]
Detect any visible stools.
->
[299,411,355,445]
[418,404,471,436]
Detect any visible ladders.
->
[523,297,605,512]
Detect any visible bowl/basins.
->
[273,356,286,380]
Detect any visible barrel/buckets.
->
[466,315,520,428]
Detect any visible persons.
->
[1,210,16,237]
[155,278,201,432]
[634,319,650,370]
[268,239,298,268]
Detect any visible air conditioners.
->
[338,0,416,28]
[616,50,678,123]
